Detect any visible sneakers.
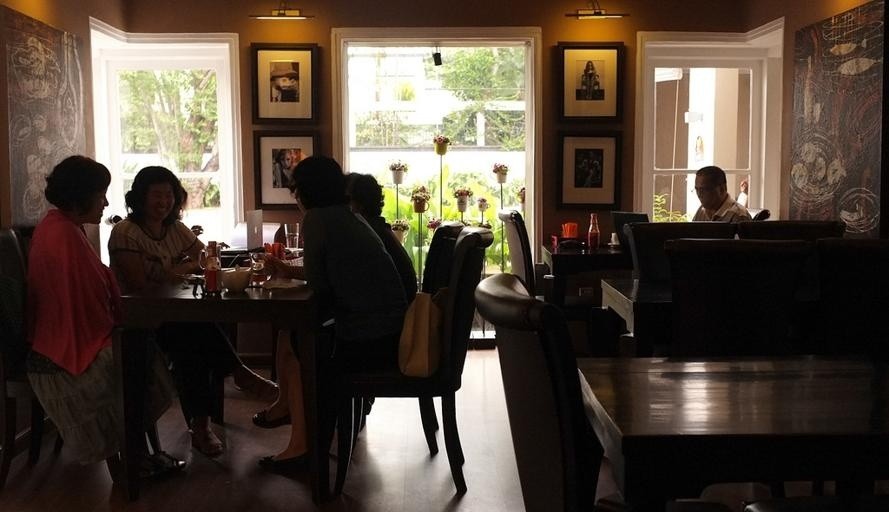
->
[191,428,226,458]
[257,449,318,467]
[233,374,279,401]
[252,407,296,430]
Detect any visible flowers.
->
[388,159,410,172]
[409,185,431,200]
[479,198,488,208]
[518,186,525,202]
[493,163,508,174]
[454,188,473,202]
[427,219,439,227]
[479,223,493,230]
[433,136,449,143]
[391,219,409,230]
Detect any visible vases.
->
[435,144,448,155]
[479,205,486,212]
[393,231,403,239]
[391,172,403,185]
[457,203,468,212]
[496,175,506,182]
[413,200,426,213]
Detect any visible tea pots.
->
[198,241,222,293]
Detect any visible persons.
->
[691,165,748,224]
[582,61,599,99]
[250,154,404,468]
[341,173,416,300]
[106,165,278,457]
[275,149,295,188]
[25,154,187,482]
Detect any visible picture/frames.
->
[557,41,625,123]
[253,131,325,210]
[554,129,621,212]
[251,41,320,127]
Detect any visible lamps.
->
[565,6,632,21]
[247,9,315,22]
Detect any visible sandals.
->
[141,460,172,483]
[152,451,187,470]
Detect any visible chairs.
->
[499,212,551,295]
[742,220,843,239]
[0,231,40,471]
[421,221,465,292]
[623,221,737,282]
[812,240,883,355]
[474,274,614,511]
[335,228,493,500]
[670,240,806,357]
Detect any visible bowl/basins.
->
[223,267,251,293]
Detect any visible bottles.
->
[204,242,221,295]
[588,213,600,251]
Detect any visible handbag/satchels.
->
[396,290,444,379]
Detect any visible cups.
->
[285,221,299,257]
[249,251,267,288]
